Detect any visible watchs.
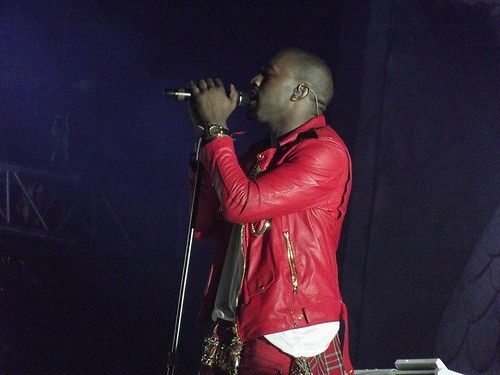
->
[202,124,232,142]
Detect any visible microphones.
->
[162,87,251,109]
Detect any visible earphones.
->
[294,89,302,96]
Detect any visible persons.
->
[187,45,353,374]
